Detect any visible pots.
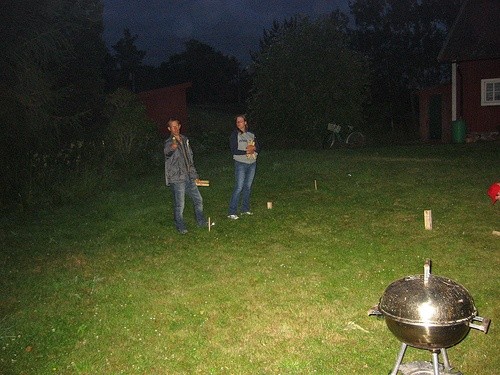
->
[367,258,491,351]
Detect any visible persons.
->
[228,113,262,219]
[164,117,214,233]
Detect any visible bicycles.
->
[323,117,365,151]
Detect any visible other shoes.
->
[227,215,240,220]
[177,230,188,234]
[205,222,215,226]
[241,211,253,216]
